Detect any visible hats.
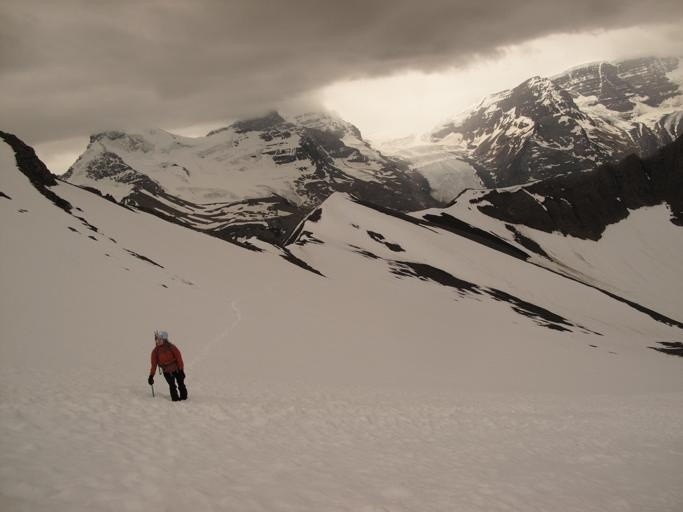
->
[153,328,168,339]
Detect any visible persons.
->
[148,329,188,401]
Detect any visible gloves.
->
[148,375,154,385]
